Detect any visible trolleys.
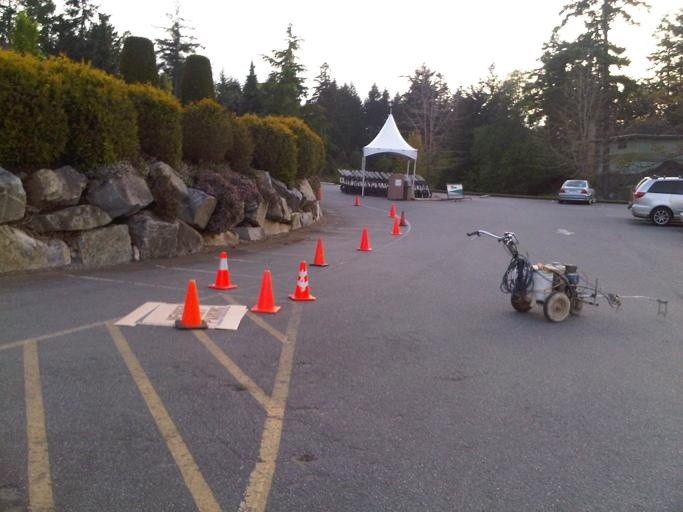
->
[464,229,668,324]
[334,168,432,199]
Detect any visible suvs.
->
[626,176,681,227]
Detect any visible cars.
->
[557,178,596,205]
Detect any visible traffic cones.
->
[172,279,208,330]
[355,228,373,253]
[310,241,331,269]
[353,193,361,207]
[287,259,318,304]
[387,201,409,236]
[208,252,237,290]
[247,268,283,316]
